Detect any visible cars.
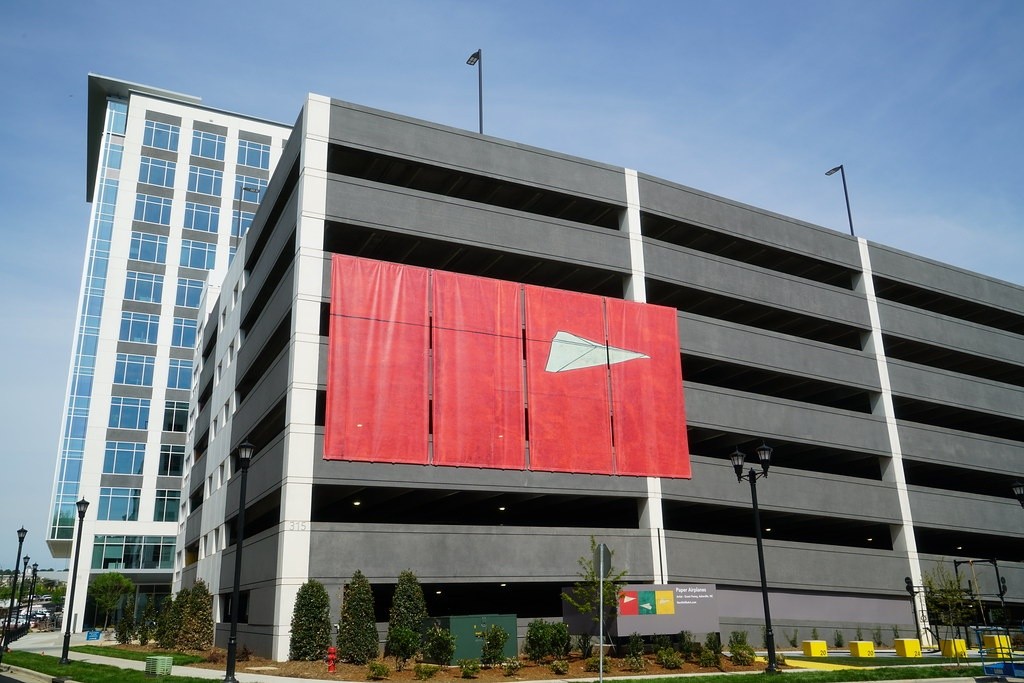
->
[0,595,52,632]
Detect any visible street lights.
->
[14,553,30,628]
[22,563,38,626]
[222,438,257,683]
[59,496,90,663]
[3,524,28,652]
[825,163,854,239]
[728,437,779,671]
[466,48,484,135]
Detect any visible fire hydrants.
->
[324,646,338,674]
